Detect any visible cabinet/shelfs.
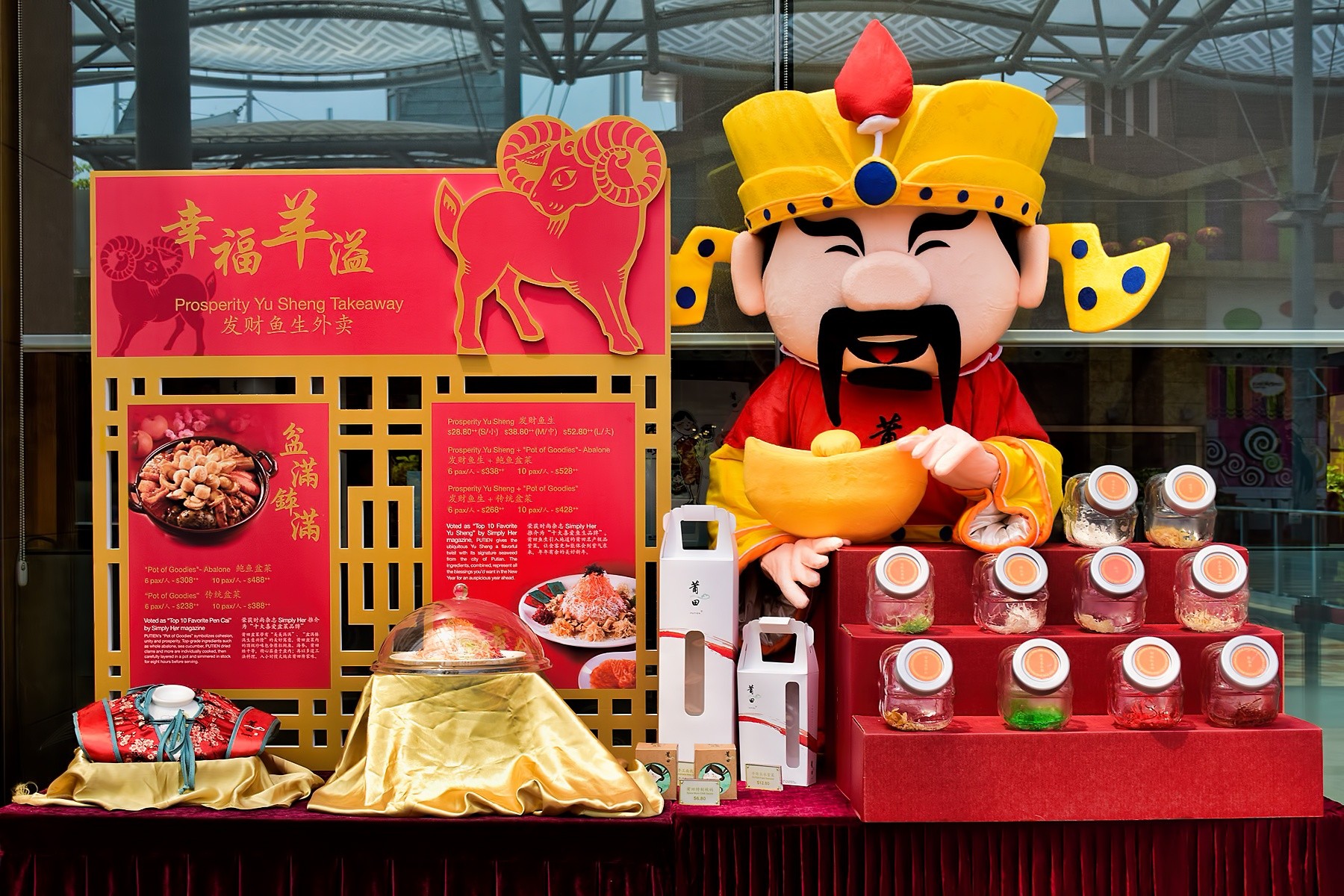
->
[812,539,1326,822]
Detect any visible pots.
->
[127,436,278,542]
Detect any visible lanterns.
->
[1129,237,1157,251]
[1195,226,1223,248]
[1103,241,1123,257]
[1163,232,1190,252]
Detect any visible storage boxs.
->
[735,616,818,788]
[694,743,738,800]
[659,504,740,786]
[635,743,679,799]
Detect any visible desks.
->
[0,783,1344,896]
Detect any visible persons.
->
[706,17,1170,657]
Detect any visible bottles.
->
[867,545,937,634]
[1076,545,1147,633]
[1145,464,1217,550]
[1106,638,1185,730]
[1061,464,1138,547]
[878,638,955,730]
[1201,635,1281,726]
[972,546,1048,633]
[996,639,1075,730]
[1174,545,1251,633]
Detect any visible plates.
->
[390,650,526,667]
[518,574,636,647]
[578,651,636,689]
